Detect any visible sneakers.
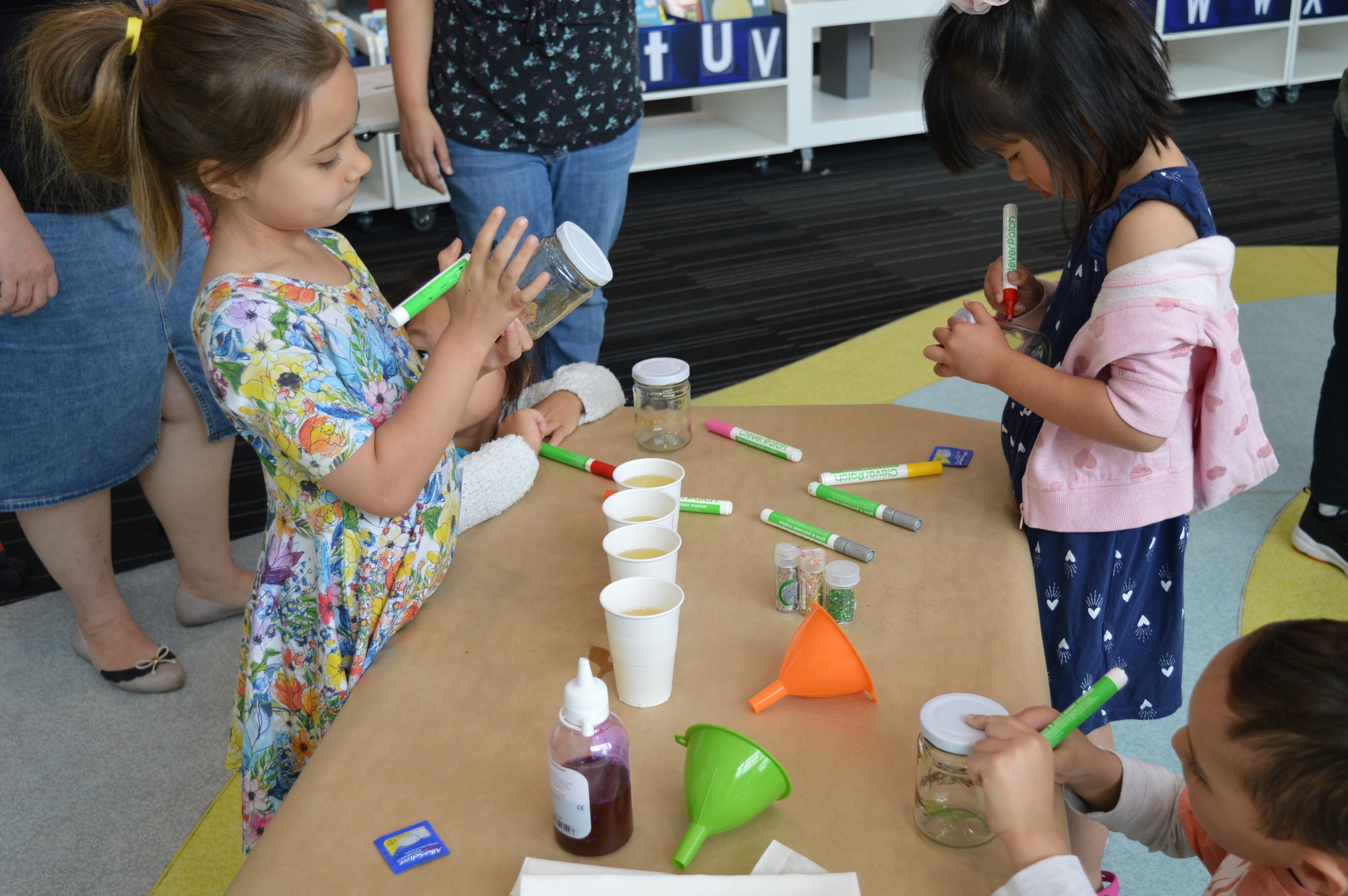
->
[1290,486,1348,579]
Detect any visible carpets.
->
[0,248,1348,896]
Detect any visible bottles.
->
[773,543,800,614]
[943,306,1052,364]
[508,220,614,343]
[824,560,860,622]
[798,546,825,618]
[913,696,1012,847]
[546,658,634,858]
[630,356,691,452]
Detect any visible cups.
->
[603,526,683,587]
[612,457,685,494]
[603,490,676,527]
[600,575,684,707]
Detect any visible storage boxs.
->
[820,22,870,98]
[1136,0,1348,34]
[638,10,787,91]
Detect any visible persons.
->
[20,0,550,854]
[393,264,626,538]
[387,0,645,386]
[965,618,1348,896]
[1291,65,1348,575]
[0,0,258,693]
[922,0,1279,896]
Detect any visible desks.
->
[224,404,1063,896]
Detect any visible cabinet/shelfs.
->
[322,11,392,237]
[1285,0,1348,103]
[327,0,1295,233]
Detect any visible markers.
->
[808,482,923,532]
[760,508,874,564]
[706,418,802,462]
[1002,203,1018,324]
[604,490,733,516]
[538,441,617,481]
[819,461,943,486]
[388,240,497,328]
[1038,665,1128,748]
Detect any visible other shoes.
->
[174,583,246,626]
[70,619,185,693]
